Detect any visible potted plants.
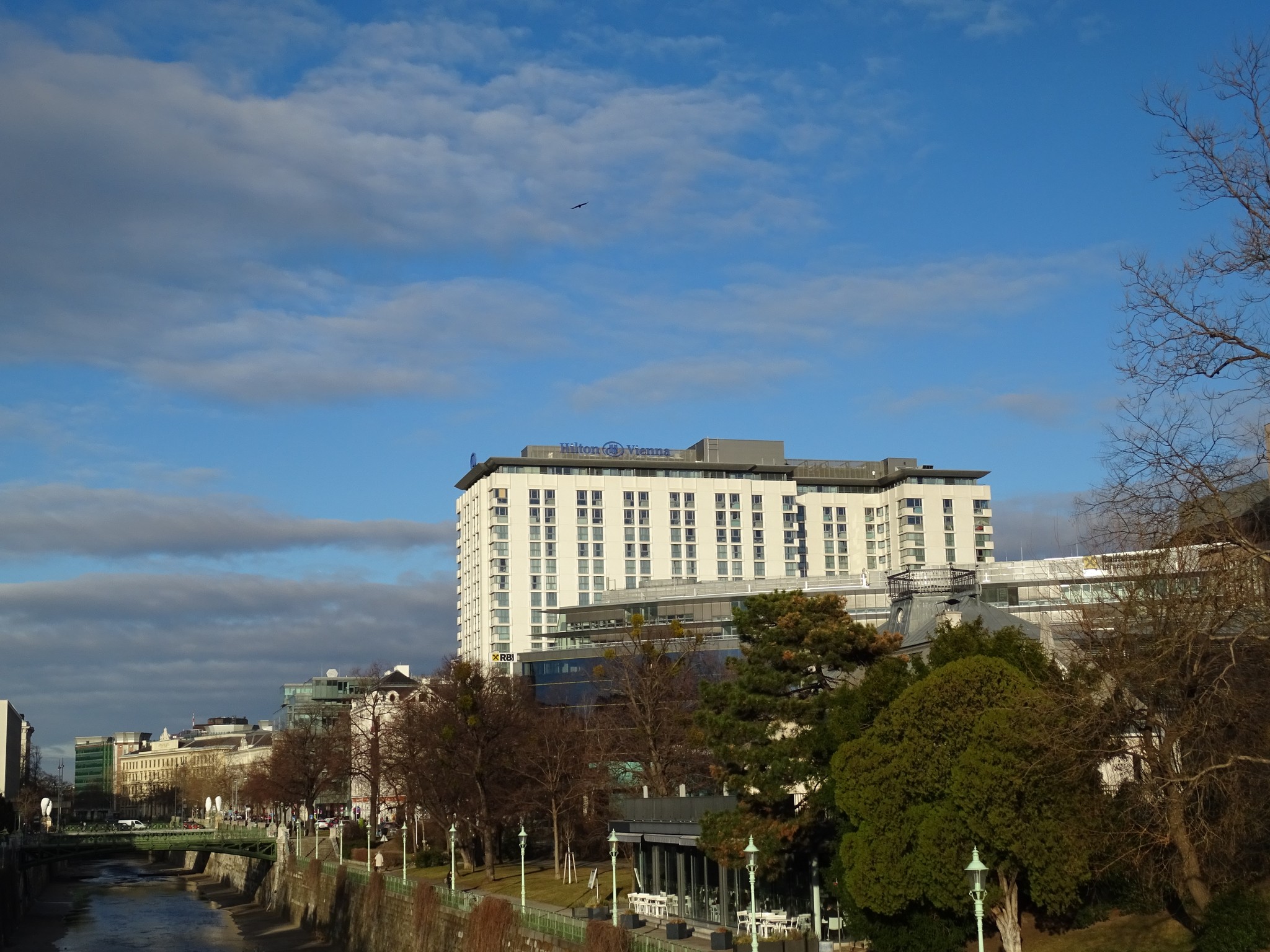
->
[621,908,640,929]
[585,895,610,921]
[732,932,819,952]
[664,916,687,939]
[709,926,732,950]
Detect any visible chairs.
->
[626,890,692,917]
[729,904,811,938]
[824,917,846,942]
[710,905,719,921]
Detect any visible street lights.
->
[607,828,620,927]
[448,822,458,896]
[743,833,760,952]
[300,799,306,837]
[279,801,285,827]
[517,825,528,914]
[234,780,238,821]
[400,821,408,885]
[964,844,990,952]
[366,820,372,876]
[338,820,345,866]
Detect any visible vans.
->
[118,820,147,830]
[112,822,131,831]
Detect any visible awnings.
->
[607,833,699,846]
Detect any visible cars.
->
[249,813,272,823]
[314,817,343,830]
[228,813,242,820]
[183,821,205,829]
[377,822,399,834]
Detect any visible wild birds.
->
[570,201,590,210]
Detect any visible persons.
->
[80,821,87,832]
[131,821,135,829]
[245,820,248,826]
[22,825,25,832]
[235,810,238,820]
[193,811,195,818]
[372,851,384,873]
[446,870,452,893]
[190,819,194,822]
[105,821,110,832]
[265,822,269,828]
[287,821,293,837]
[300,820,303,828]
